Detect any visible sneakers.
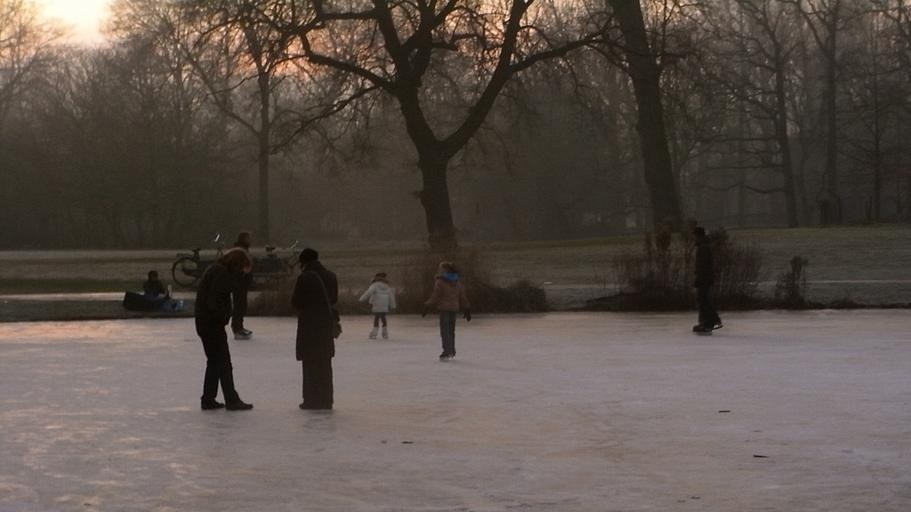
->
[234,327,252,337]
[225,398,253,410]
[439,351,454,358]
[692,324,713,333]
[200,398,224,410]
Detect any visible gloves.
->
[421,305,430,318]
[463,309,472,322]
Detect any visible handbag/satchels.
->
[329,308,343,339]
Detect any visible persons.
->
[143,271,164,297]
[231,232,251,336]
[195,248,251,411]
[422,260,472,357]
[292,248,337,410]
[213,230,223,255]
[690,226,720,332]
[358,273,396,337]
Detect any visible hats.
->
[691,226,708,235]
[298,248,318,264]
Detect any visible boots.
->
[369,327,379,336]
[381,327,389,337]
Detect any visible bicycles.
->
[172,232,226,288]
[258,242,305,282]
